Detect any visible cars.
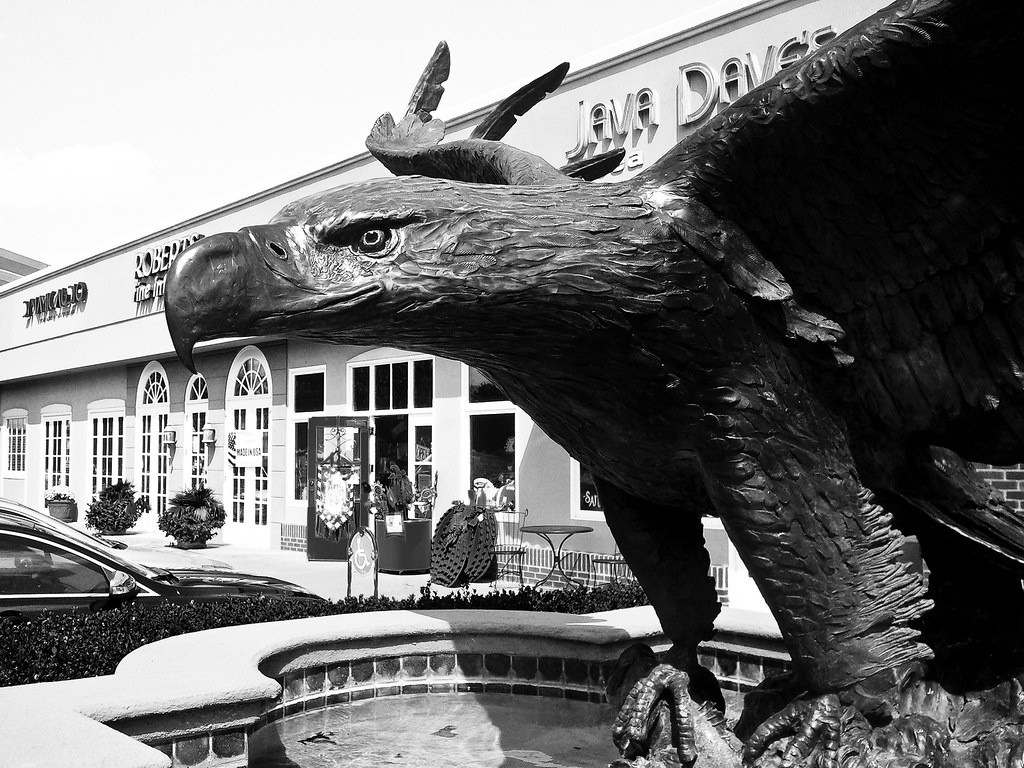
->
[0,496,327,626]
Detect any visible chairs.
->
[483,507,529,592]
[591,543,634,587]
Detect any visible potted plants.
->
[45,492,78,523]
[157,481,227,548]
[85,478,151,535]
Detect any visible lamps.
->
[201,422,217,443]
[162,425,177,444]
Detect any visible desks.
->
[520,526,593,591]
